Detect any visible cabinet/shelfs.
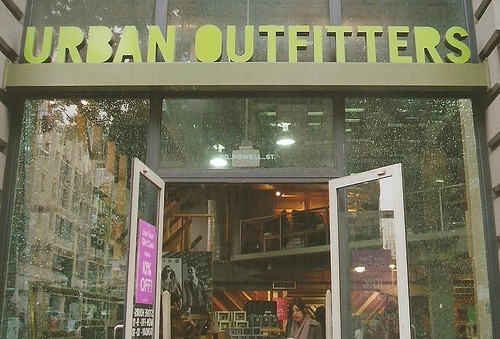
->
[16,105,125,339]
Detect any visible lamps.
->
[275,125,296,146]
[208,145,229,168]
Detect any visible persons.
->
[166,292,196,339]
[284,296,324,339]
[183,262,211,339]
[161,265,183,300]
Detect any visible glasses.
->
[165,269,171,272]
